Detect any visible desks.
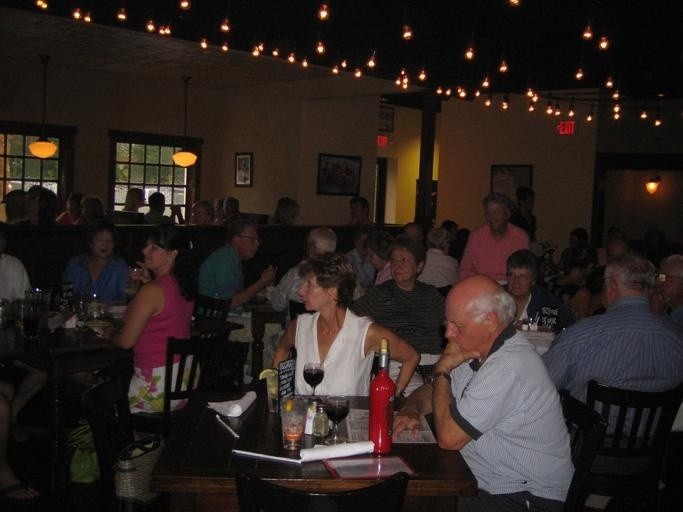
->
[146,398,479,505]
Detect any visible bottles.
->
[367,336,394,455]
[303,395,321,435]
[312,404,328,436]
[521,317,534,331]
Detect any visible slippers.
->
[0,482,42,506]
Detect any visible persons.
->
[1,187,682,512]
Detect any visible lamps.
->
[25,53,58,159]
[172,75,199,167]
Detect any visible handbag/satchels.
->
[112,433,164,506]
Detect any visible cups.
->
[18,287,103,339]
[264,292,272,301]
[126,264,143,286]
[279,396,307,450]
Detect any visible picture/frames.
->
[315,152,362,196]
[377,105,394,133]
[234,151,252,188]
[487,164,532,194]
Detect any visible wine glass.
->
[302,363,325,402]
[324,396,350,445]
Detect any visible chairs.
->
[2,205,681,389]
[585,376,683,511]
[128,338,208,448]
[232,470,408,510]
[558,390,608,511]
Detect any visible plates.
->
[107,305,128,314]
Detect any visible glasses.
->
[236,234,263,244]
[506,271,536,282]
